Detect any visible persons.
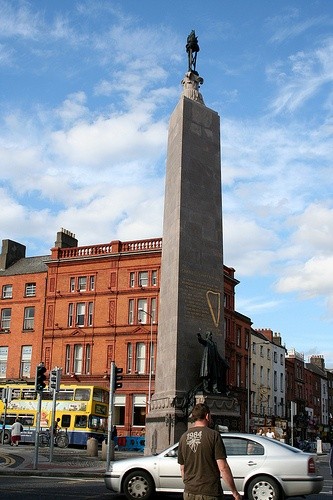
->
[256,428,276,440]
[10,418,22,445]
[113,425,117,444]
[178,403,241,500]
[79,416,85,426]
[48,418,61,446]
[197,331,223,394]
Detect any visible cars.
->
[105,433,324,500]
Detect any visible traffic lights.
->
[51,371,57,388]
[38,367,47,389]
[115,366,123,390]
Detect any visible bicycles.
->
[39,428,69,448]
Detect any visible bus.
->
[0,380,107,447]
